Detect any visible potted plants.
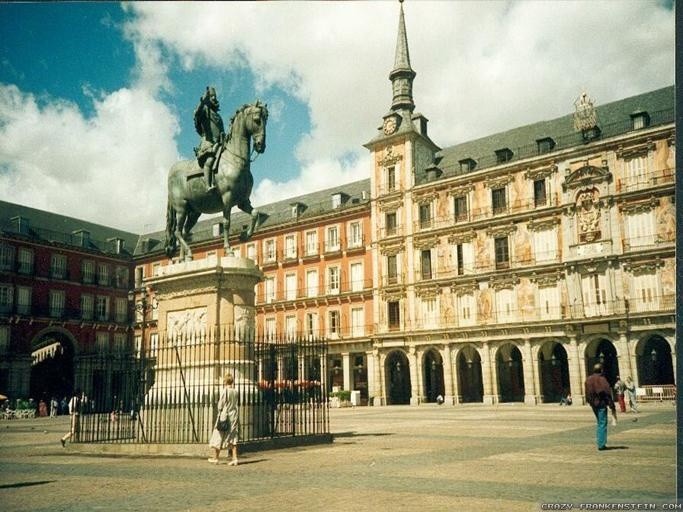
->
[328,391,339,408]
[338,390,353,408]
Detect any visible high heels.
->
[208,457,220,465]
[228,459,239,466]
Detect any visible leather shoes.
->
[60,438,66,447]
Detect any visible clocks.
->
[382,116,397,135]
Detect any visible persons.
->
[613,375,627,412]
[108,393,124,423]
[559,393,572,406]
[584,362,617,450]
[624,375,637,412]
[0,395,59,419]
[206,374,240,466]
[191,85,229,194]
[60,396,68,415]
[563,265,581,305]
[603,259,622,304]
[59,391,82,447]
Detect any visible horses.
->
[163,98,271,263]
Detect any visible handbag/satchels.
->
[216,415,230,432]
